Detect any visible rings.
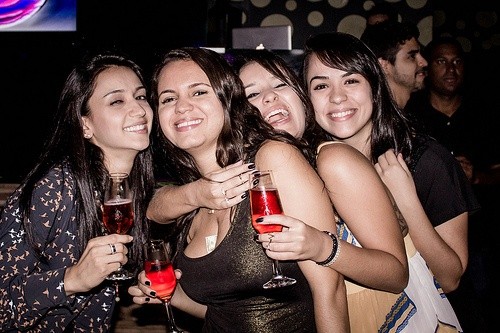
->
[237,175,243,184]
[107,242,115,255]
[265,243,271,251]
[268,232,274,243]
[223,190,229,202]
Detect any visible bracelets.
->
[315,229,341,267]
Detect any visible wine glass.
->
[103,172,136,280]
[142,240,188,333]
[249,170,297,289]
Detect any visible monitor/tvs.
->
[232,25,292,50]
[0,0,77,32]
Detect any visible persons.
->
[143,48,463,333]
[0,53,161,332]
[127,45,318,333]
[421,36,490,262]
[300,32,471,333]
[359,19,430,117]
